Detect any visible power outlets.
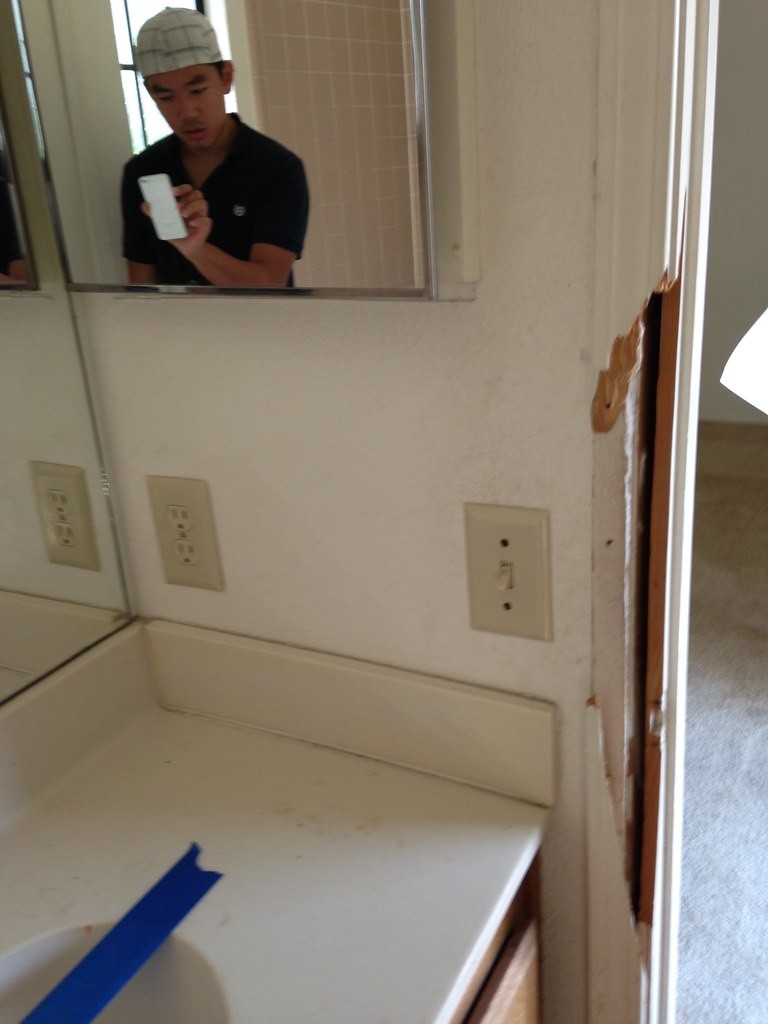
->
[147,474,223,592]
[29,458,99,571]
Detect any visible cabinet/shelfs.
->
[463,852,544,1022]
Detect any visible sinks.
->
[0,921,230,1023]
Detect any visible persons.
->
[120,8,308,288]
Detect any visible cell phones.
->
[138,173,188,240]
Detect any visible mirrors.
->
[0,104,39,291]
[1,0,137,744]
[14,0,437,307]
